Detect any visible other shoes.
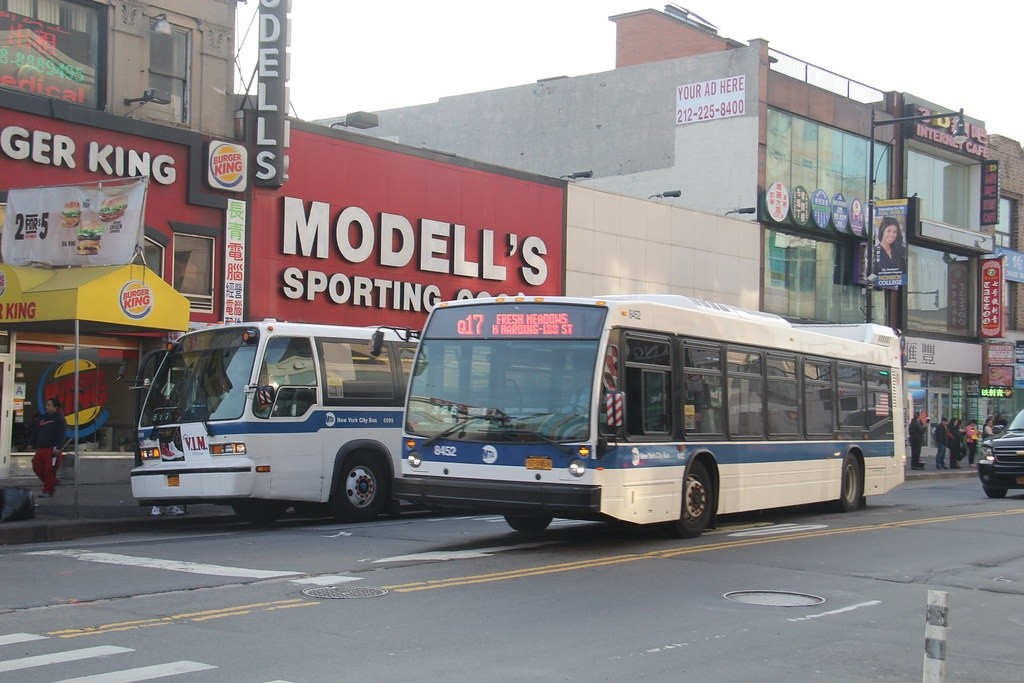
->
[38,490,54,498]
[968,463,977,467]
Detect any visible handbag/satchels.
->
[946,431,954,441]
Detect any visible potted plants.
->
[117,436,131,452]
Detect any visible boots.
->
[160,442,176,460]
[167,441,184,459]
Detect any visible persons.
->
[873,218,905,274]
[944,417,964,469]
[908,412,931,470]
[957,420,965,448]
[150,504,186,516]
[966,418,980,467]
[981,419,994,442]
[32,398,65,500]
[934,418,949,470]
[560,367,591,415]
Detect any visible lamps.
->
[329,111,378,129]
[725,208,756,215]
[123,88,171,104]
[648,190,681,200]
[558,170,593,179]
[151,13,172,36]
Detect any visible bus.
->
[115,318,420,526]
[367,292,907,540]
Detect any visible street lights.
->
[865,102,967,323]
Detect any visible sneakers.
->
[165,505,185,516]
[150,505,162,516]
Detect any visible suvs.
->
[977,406,1024,499]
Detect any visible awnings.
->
[0,263,190,488]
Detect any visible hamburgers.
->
[61,196,128,255]
